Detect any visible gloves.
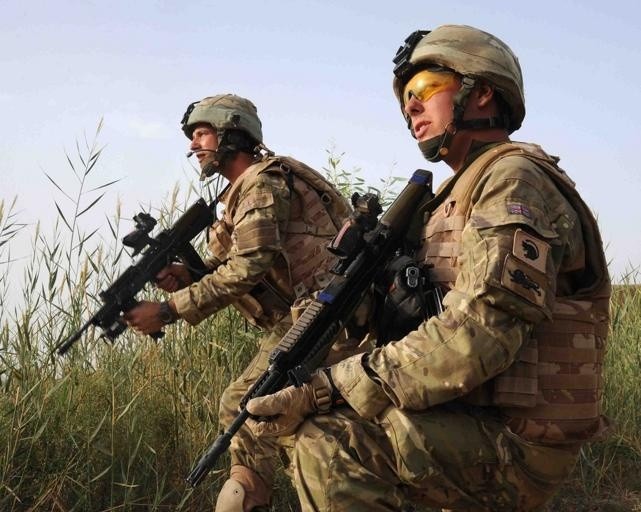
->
[237,366,334,439]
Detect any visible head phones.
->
[218,136,239,159]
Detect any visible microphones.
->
[186,149,217,159]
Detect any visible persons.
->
[248,23,611,512]
[120,92,356,511]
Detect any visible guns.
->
[57,197,215,355]
[187,169,436,487]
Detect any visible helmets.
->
[392,24,525,135]
[180,94,263,143]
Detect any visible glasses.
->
[402,68,461,109]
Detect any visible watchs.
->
[158,301,175,323]
